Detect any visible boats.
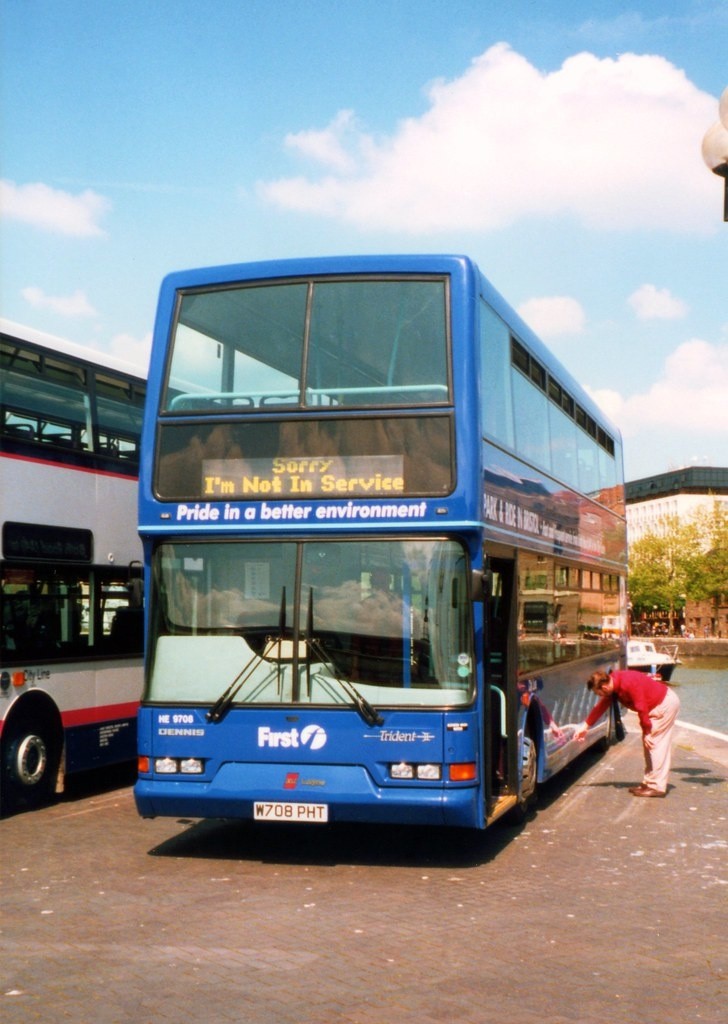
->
[625,639,681,683]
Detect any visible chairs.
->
[109,605,143,653]
[259,393,310,407]
[0,423,135,461]
[209,395,254,410]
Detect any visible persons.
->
[518,671,567,745]
[573,670,681,797]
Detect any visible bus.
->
[0,317,406,813]
[137,253,630,830]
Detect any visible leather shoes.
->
[629,784,665,798]
